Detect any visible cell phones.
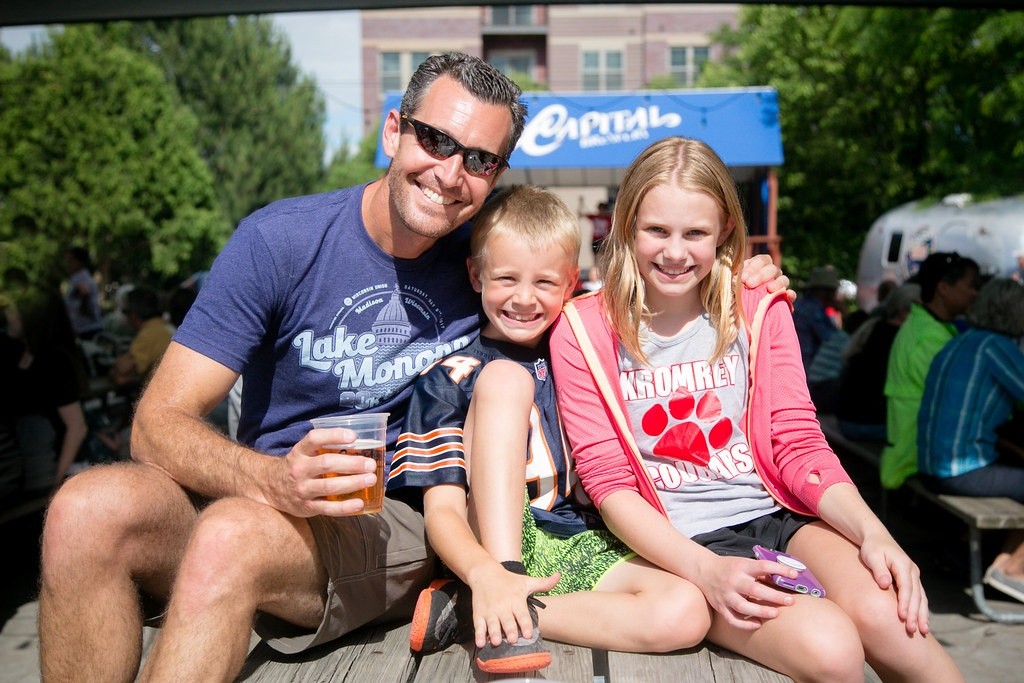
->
[753,544,826,599]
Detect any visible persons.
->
[0,247,195,486]
[790,250,1024,601]
[578,195,614,266]
[39,52,968,683]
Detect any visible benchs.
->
[817,413,1024,625]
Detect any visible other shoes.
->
[983,568,1024,605]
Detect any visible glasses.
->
[400,112,511,176]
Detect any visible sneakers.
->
[476,598,552,674]
[410,578,474,655]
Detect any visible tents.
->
[374,85,785,270]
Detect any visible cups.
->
[308,413,392,517]
